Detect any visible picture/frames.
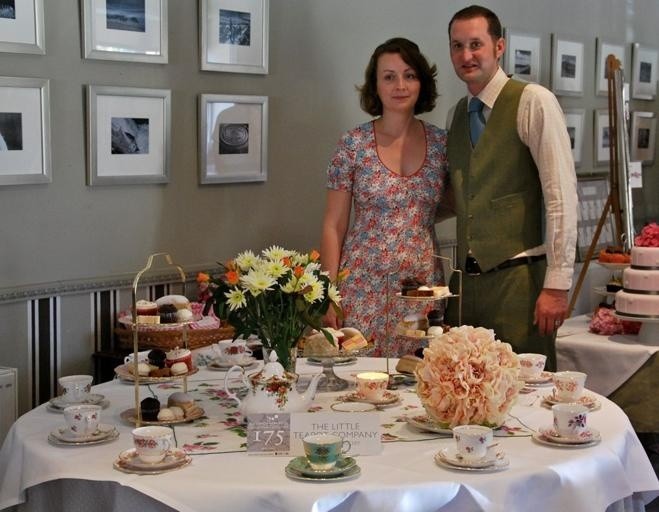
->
[198,0,270,75]
[86,85,173,186]
[0,0,46,57]
[197,92,270,185]
[0,76,52,188]
[505,27,658,172]
[79,0,170,63]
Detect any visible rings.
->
[554,320,561,325]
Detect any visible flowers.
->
[197,244,354,356]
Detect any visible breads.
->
[302,326,368,357]
[396,275,452,375]
[127,294,205,422]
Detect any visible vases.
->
[264,345,296,374]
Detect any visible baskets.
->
[115,320,235,351]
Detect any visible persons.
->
[320,38,449,361]
[435,7,579,375]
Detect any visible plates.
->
[595,287,618,295]
[342,391,400,407]
[595,260,632,270]
[409,408,505,434]
[209,358,258,370]
[287,458,364,481]
[542,391,602,414]
[302,352,359,368]
[436,449,513,472]
[531,425,605,448]
[115,362,199,383]
[115,449,193,474]
[331,400,377,413]
[517,372,553,385]
[393,288,452,300]
[120,404,205,426]
[47,424,121,445]
[117,302,208,333]
[49,394,110,412]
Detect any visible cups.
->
[551,369,587,400]
[514,352,548,376]
[212,338,251,364]
[131,424,175,462]
[552,402,591,436]
[356,372,391,398]
[63,406,103,436]
[302,434,350,469]
[57,374,93,403]
[452,426,500,459]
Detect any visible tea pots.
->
[224,348,326,414]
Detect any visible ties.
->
[469,97,485,147]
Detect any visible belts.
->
[466,255,546,276]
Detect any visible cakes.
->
[615,224,659,315]
